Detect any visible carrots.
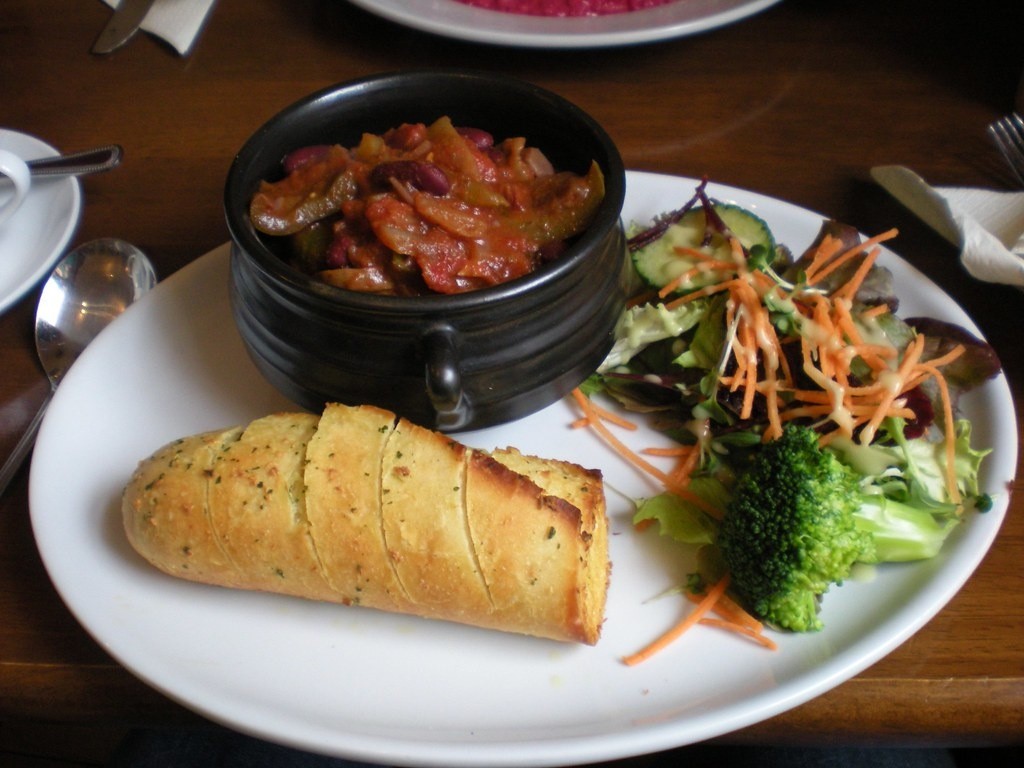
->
[574,230,964,665]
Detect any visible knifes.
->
[91,0,156,54]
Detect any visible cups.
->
[0,147,32,225]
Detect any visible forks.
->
[986,112,1024,187]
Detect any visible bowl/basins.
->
[220,63,634,435]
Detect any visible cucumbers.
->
[633,202,773,292]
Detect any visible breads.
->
[119,404,608,644]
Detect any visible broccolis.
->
[718,427,959,633]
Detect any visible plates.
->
[27,170,1019,768]
[0,128,81,316]
[348,0,783,48]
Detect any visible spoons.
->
[0,236,158,496]
